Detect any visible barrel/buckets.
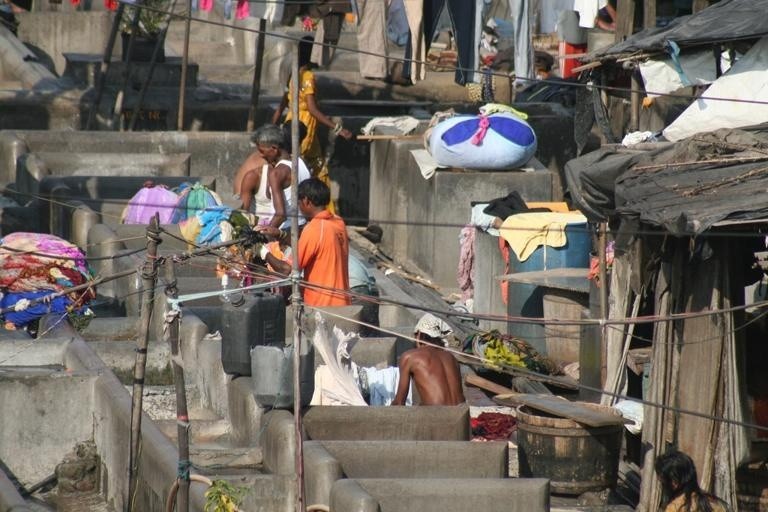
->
[508,214,592,362]
[471,221,508,339]
[543,286,588,374]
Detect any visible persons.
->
[255,179,351,306]
[232,120,308,195]
[248,126,313,232]
[534,48,557,86]
[271,36,354,216]
[593,1,620,32]
[389,313,466,407]
[239,160,287,227]
[653,451,732,512]
